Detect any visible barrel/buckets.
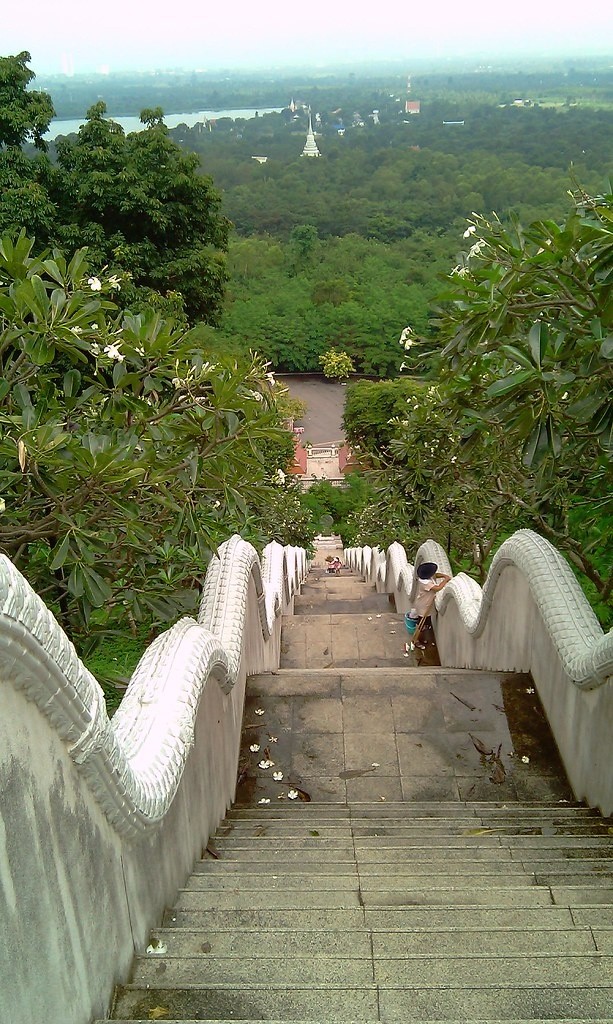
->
[405,611,417,634]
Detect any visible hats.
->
[417,562,438,580]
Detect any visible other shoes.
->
[414,642,425,649]
[420,639,427,644]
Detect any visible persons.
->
[325,557,341,577]
[412,563,450,649]
